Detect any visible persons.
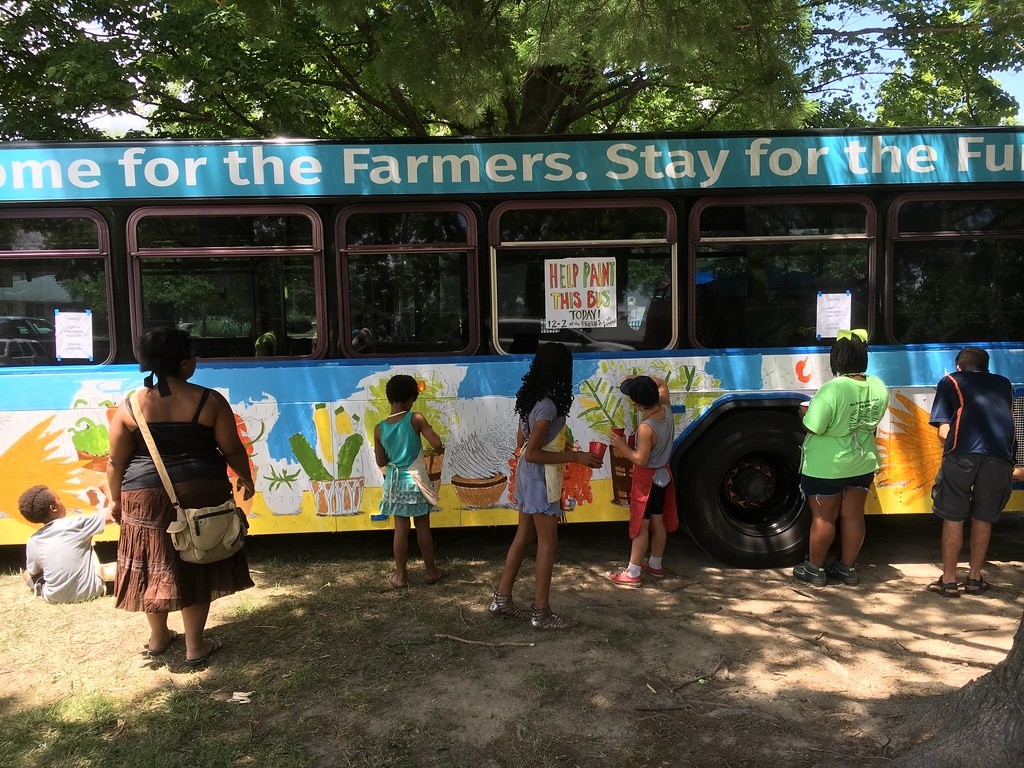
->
[926,347,1018,598]
[107,327,256,666]
[601,374,678,588]
[488,342,603,631]
[793,329,889,587]
[373,375,449,589]
[18,484,115,604]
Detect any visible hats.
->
[620,376,659,405]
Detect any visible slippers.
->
[388,572,407,588]
[149,629,177,655]
[184,638,222,663]
[425,569,449,584]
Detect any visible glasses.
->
[192,352,200,360]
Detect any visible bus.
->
[0,126,1024,569]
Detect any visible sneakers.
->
[640,559,665,578]
[603,569,642,587]
[825,553,858,587]
[793,555,827,587]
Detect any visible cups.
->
[611,427,625,437]
[588,442,608,459]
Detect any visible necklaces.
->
[645,406,662,421]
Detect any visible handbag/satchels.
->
[166,497,250,564]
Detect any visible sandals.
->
[926,574,960,598]
[488,590,531,621]
[530,604,579,630]
[965,575,989,596]
